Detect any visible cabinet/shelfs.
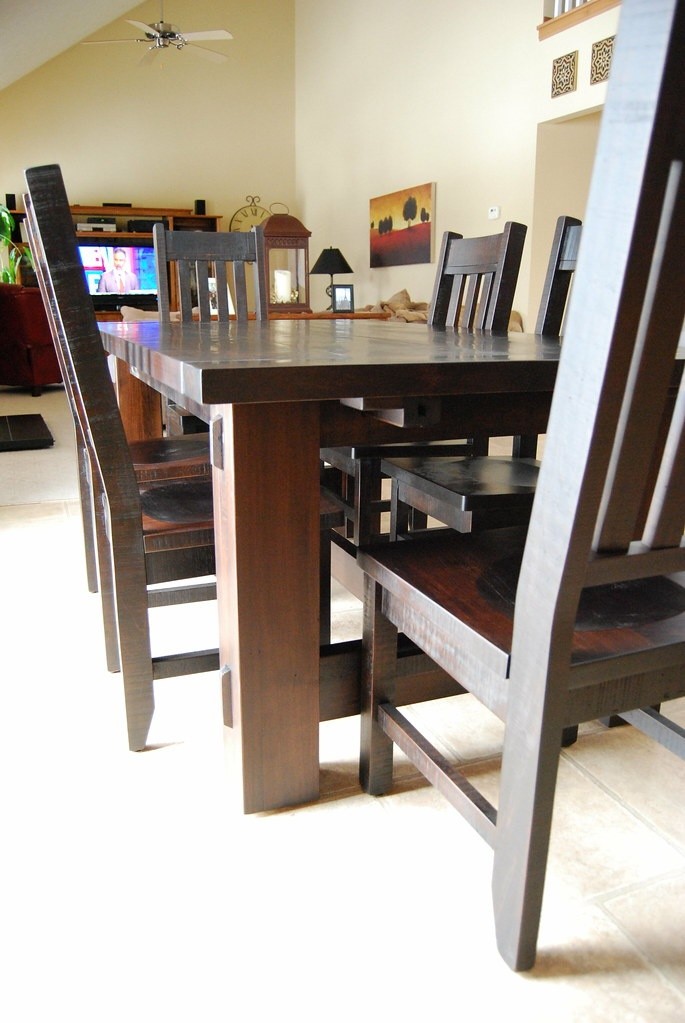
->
[5,192,224,399]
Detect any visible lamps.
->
[309,246,354,311]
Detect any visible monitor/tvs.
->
[77,236,172,313]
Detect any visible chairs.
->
[324,222,527,551]
[22,162,329,746]
[384,217,584,540]
[354,0,685,970]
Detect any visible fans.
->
[81,0,233,65]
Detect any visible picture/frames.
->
[331,285,354,313]
[208,277,236,316]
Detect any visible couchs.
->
[358,299,523,331]
[0,283,63,397]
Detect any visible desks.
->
[95,319,685,814]
[172,310,393,323]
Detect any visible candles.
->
[273,270,292,304]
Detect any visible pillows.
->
[383,288,412,313]
[118,304,161,322]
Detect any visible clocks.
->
[230,195,273,265]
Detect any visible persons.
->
[97,248,139,293]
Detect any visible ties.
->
[119,274,125,293]
